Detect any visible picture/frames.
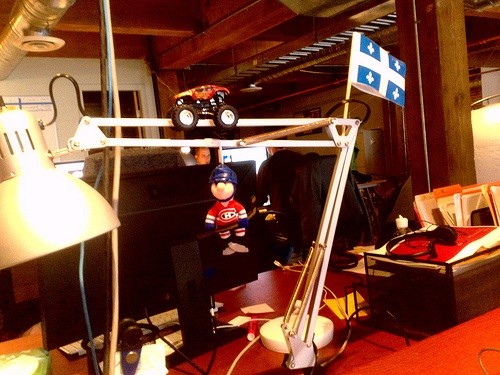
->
[293,107,323,137]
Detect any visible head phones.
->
[387,225,456,259]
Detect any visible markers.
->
[246,316,257,341]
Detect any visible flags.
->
[349,32,407,108]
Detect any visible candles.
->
[395,215,409,228]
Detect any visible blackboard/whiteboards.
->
[1,95,61,163]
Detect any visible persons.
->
[195,147,211,165]
[205,164,249,256]
[251,126,304,259]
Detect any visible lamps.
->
[240,74,262,93]
[0,74,363,375]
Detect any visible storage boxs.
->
[364,227,500,340]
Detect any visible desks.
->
[0,223,500,375]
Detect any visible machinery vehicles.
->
[166,84,240,132]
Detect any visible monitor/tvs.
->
[38,161,258,351]
